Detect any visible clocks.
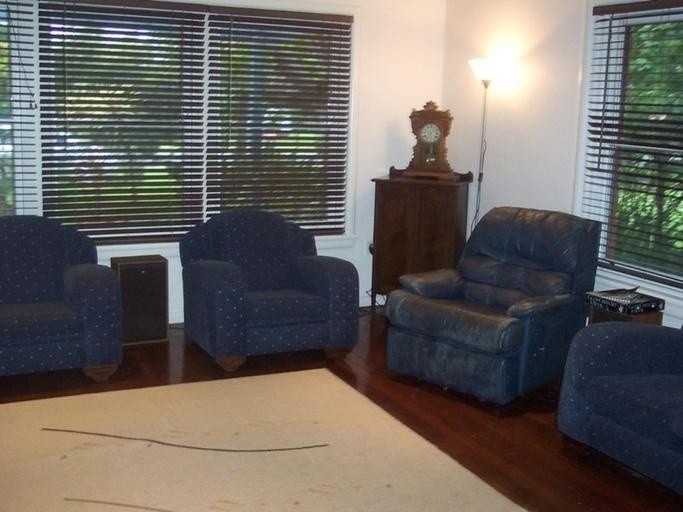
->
[404,100,455,179]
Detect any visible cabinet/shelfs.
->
[371,178,469,310]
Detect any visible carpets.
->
[0,369,536,510]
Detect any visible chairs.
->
[556,321,683,500]
[180,208,359,373]
[385,207,601,405]
[0,215,125,383]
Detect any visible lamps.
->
[468,56,504,230]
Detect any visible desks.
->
[586,289,662,326]
[108,255,168,347]
